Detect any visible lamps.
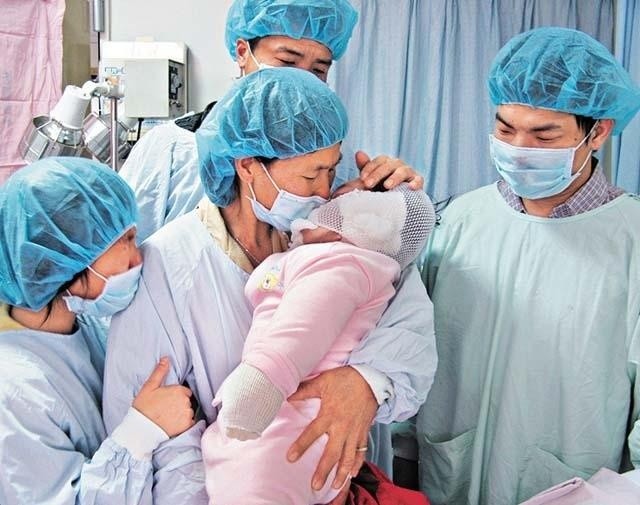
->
[18,73,132,173]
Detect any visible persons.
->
[116,0,425,251]
[101,67,439,505]
[411,27,640,505]
[197,175,438,505]
[0,156,196,505]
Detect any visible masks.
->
[246,41,335,94]
[63,264,142,319]
[248,165,330,232]
[488,122,596,200]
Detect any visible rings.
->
[356,446,369,452]
